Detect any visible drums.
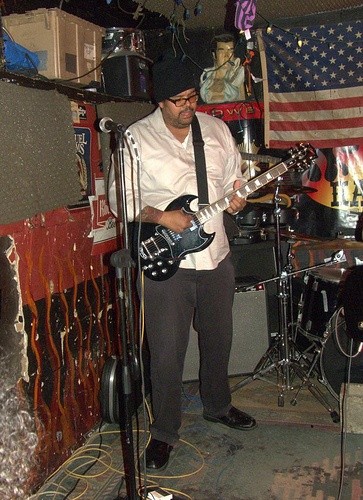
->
[292,267,348,359]
[318,304,363,402]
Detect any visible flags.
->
[256,19,363,150]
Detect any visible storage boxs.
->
[101,53,154,101]
[0,7,105,85]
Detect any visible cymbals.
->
[256,181,318,196]
[289,238,363,251]
[262,227,323,244]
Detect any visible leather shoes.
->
[143,439,173,473]
[202,405,257,431]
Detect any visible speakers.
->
[181,283,272,383]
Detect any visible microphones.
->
[99,117,122,133]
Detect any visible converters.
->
[148,489,173,500]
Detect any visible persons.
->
[200,31,246,104]
[107,59,258,472]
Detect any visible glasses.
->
[167,89,200,107]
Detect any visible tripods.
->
[230,183,341,424]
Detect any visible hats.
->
[151,62,196,105]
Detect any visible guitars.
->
[124,140,319,283]
